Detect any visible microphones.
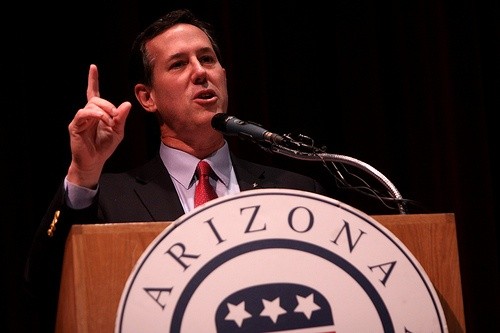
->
[211,113,287,144]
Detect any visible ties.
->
[194,160,219,210]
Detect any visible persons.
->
[31,9,324,301]
